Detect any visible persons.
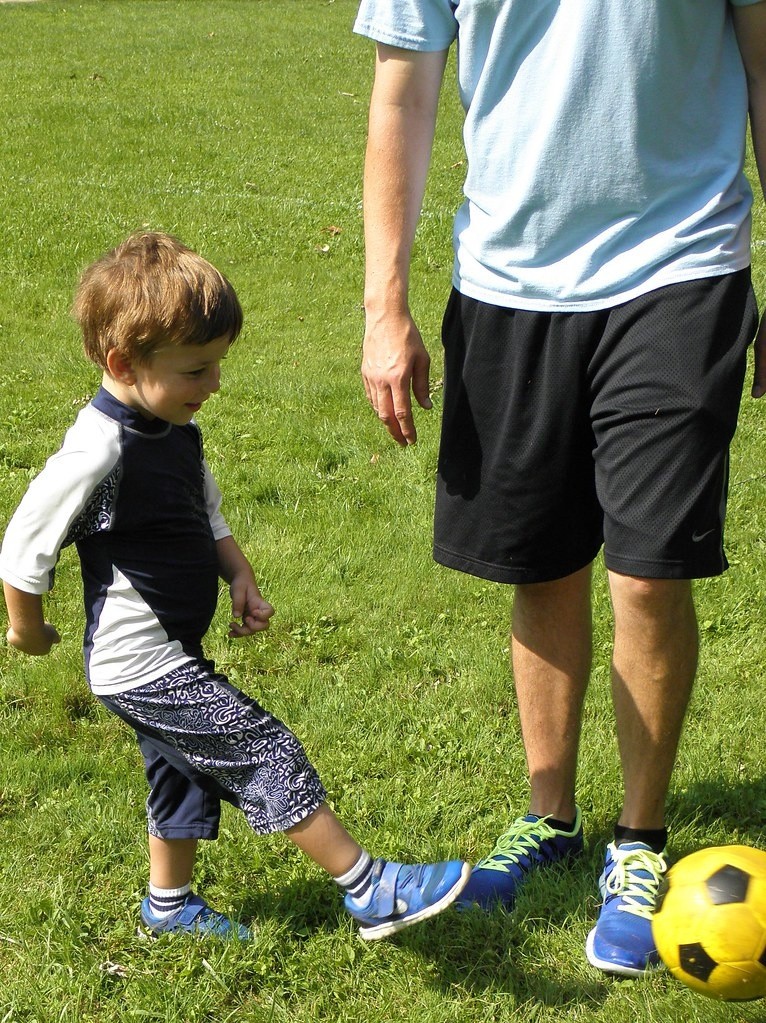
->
[354,0,766,980]
[0,234,472,939]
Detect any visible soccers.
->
[648,842,766,1006]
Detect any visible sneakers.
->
[345,859,472,940]
[588,841,669,977]
[466,801,591,914]
[136,893,252,946]
